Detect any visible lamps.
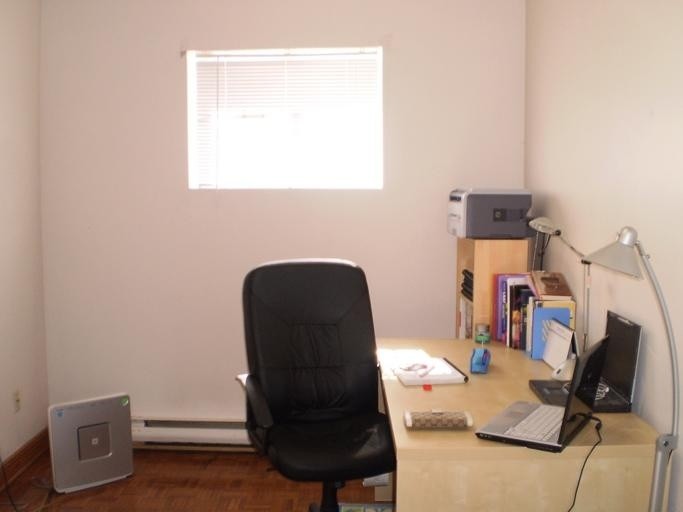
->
[527,213,592,352]
[581,226,680,512]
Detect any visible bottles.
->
[475,325,489,344]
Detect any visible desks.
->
[373,336,671,512]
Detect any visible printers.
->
[446,186,533,240]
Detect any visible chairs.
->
[234,257,395,510]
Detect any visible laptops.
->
[530,309,644,412]
[478,334,612,452]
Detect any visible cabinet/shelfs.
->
[453,238,534,340]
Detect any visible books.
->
[395,355,468,388]
[492,269,576,362]
